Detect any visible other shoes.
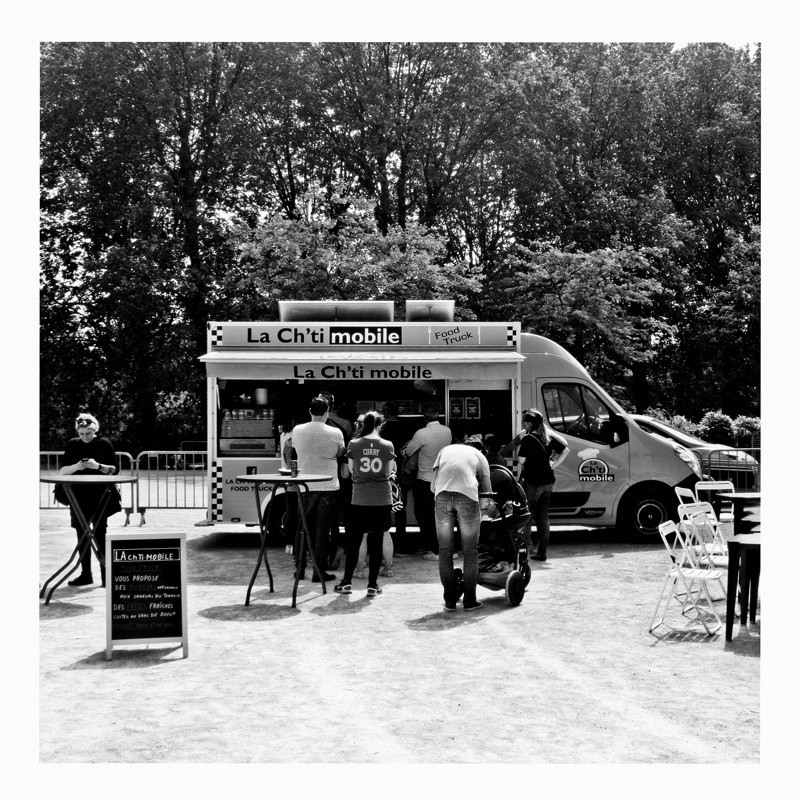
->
[312,572,336,581]
[294,570,305,580]
[352,571,367,579]
[68,575,93,586]
[530,554,546,561]
[379,569,393,576]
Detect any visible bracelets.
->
[97,465,101,470]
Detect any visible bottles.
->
[224,408,274,420]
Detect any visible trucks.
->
[198,301,721,544]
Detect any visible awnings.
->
[197,350,527,409]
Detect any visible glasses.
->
[76,419,95,424]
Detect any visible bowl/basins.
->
[279,468,300,475]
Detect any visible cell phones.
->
[82,458,88,462]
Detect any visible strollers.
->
[451,464,532,606]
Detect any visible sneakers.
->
[424,551,440,560]
[444,602,456,611]
[464,601,483,610]
[367,586,382,597]
[334,584,352,593]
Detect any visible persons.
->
[430,434,492,611]
[483,434,506,466]
[58,413,122,586]
[285,390,353,583]
[406,400,458,561]
[500,408,570,561]
[334,401,412,596]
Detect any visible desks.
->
[39,471,138,606]
[714,491,761,644]
[236,471,333,609]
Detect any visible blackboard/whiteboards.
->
[105,533,188,646]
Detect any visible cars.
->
[160,456,206,470]
[549,413,758,491]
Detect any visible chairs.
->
[648,482,735,636]
